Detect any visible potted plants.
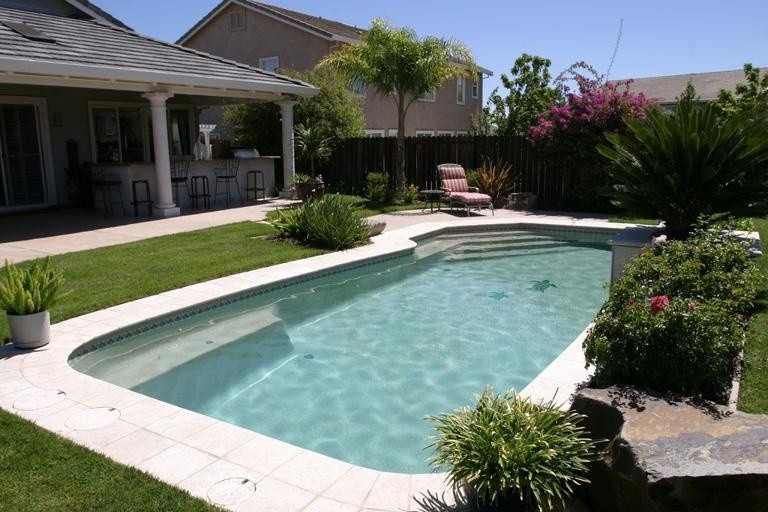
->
[294,122,337,201]
[0,255,65,350]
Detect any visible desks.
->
[419,189,445,214]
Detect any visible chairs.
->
[169,158,193,208]
[437,162,495,217]
[214,155,243,209]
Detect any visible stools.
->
[246,171,266,202]
[130,179,154,218]
[190,175,211,210]
[91,180,126,218]
